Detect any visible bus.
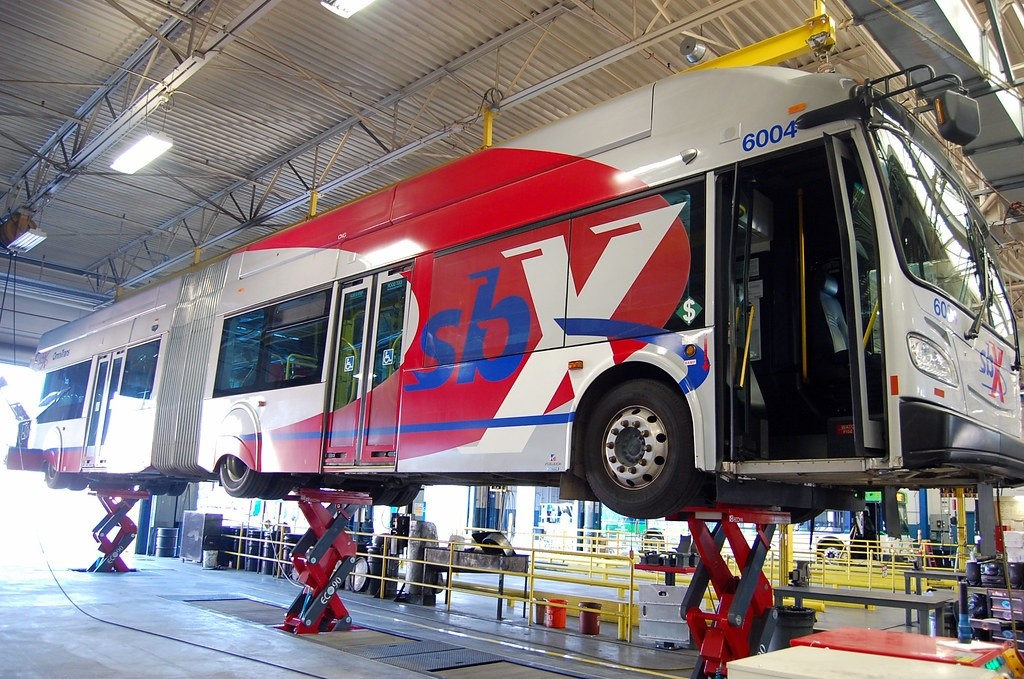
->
[601,490,910,565]
[6,64,1024,520]
